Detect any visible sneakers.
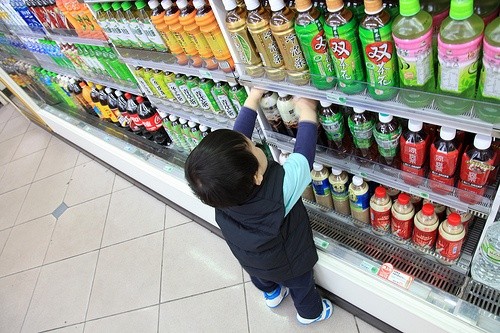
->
[297,299,333,325]
[264,286,290,307]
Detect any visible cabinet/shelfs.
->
[0,0,500,333]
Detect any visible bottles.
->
[0,0,500,290]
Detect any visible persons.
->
[185,87,334,325]
